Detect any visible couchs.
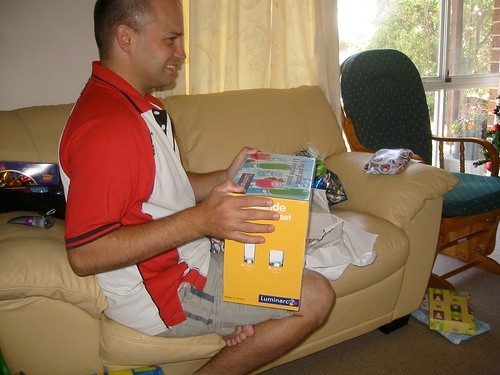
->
[0,85,459,375]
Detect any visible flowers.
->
[472,95,500,171]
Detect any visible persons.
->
[58,0,336,375]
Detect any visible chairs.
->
[340,49,500,289]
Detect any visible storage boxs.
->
[427,287,477,336]
[219,152,316,311]
[0,160,62,194]
